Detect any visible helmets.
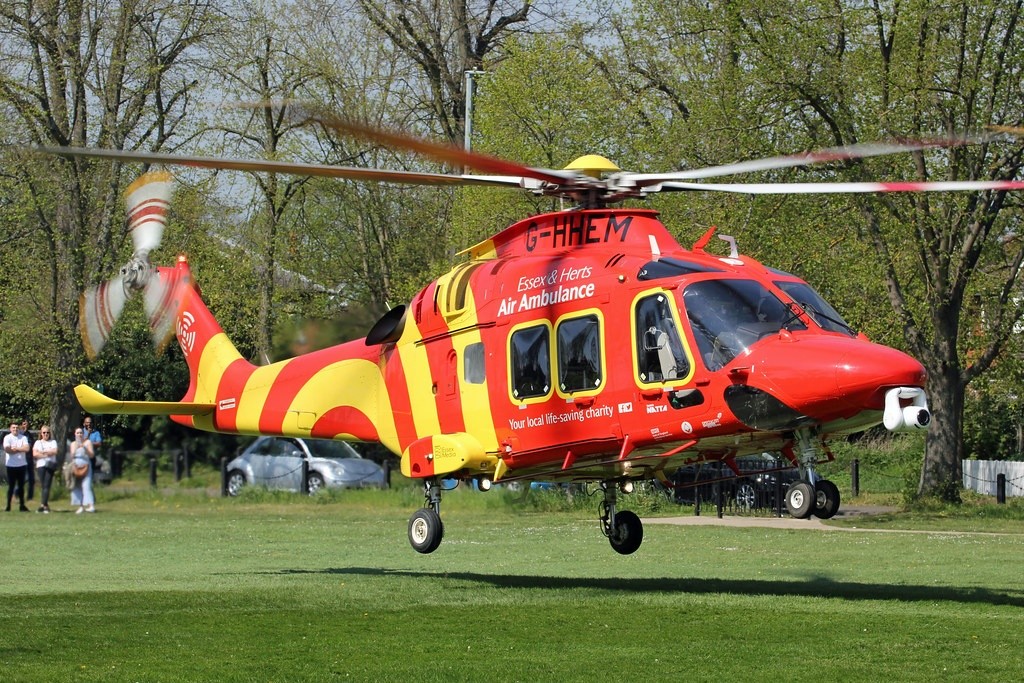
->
[705,286,753,305]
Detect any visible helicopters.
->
[45,96,1023,552]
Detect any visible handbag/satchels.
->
[72,462,89,479]
[45,461,57,472]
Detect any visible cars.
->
[666,451,822,511]
[225,435,385,495]
[0,429,112,485]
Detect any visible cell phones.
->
[89,424,93,430]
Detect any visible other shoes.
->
[36,507,50,513]
[85,506,96,513]
[76,507,84,514]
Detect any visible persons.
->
[82,417,102,479]
[65,428,96,514]
[32,425,57,513]
[3,422,30,511]
[15,419,34,501]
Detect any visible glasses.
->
[43,432,50,434]
